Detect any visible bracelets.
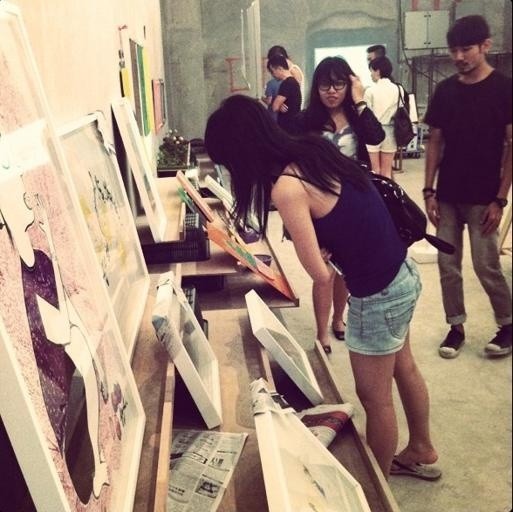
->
[423,188,435,194]
[424,195,433,199]
[356,100,367,107]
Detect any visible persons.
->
[366,45,396,82]
[423,15,512,358]
[266,54,302,210]
[202,94,443,481]
[268,47,305,110]
[298,57,389,353]
[262,77,282,118]
[362,56,402,181]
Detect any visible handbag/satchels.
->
[370,173,427,246]
[395,107,414,147]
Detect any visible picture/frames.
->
[118,25,150,217]
[255,377,373,510]
[207,223,292,300]
[49,109,148,366]
[109,100,165,243]
[154,78,165,130]
[153,269,221,430]
[244,287,326,405]
[2,120,148,512]
[176,172,217,223]
[206,177,262,231]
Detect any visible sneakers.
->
[439,326,464,358]
[485,326,513,356]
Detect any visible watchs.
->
[493,196,507,208]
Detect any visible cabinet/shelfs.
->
[132,262,405,512]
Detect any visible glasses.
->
[319,80,346,90]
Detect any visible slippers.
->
[390,456,441,479]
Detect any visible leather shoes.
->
[332,322,346,339]
